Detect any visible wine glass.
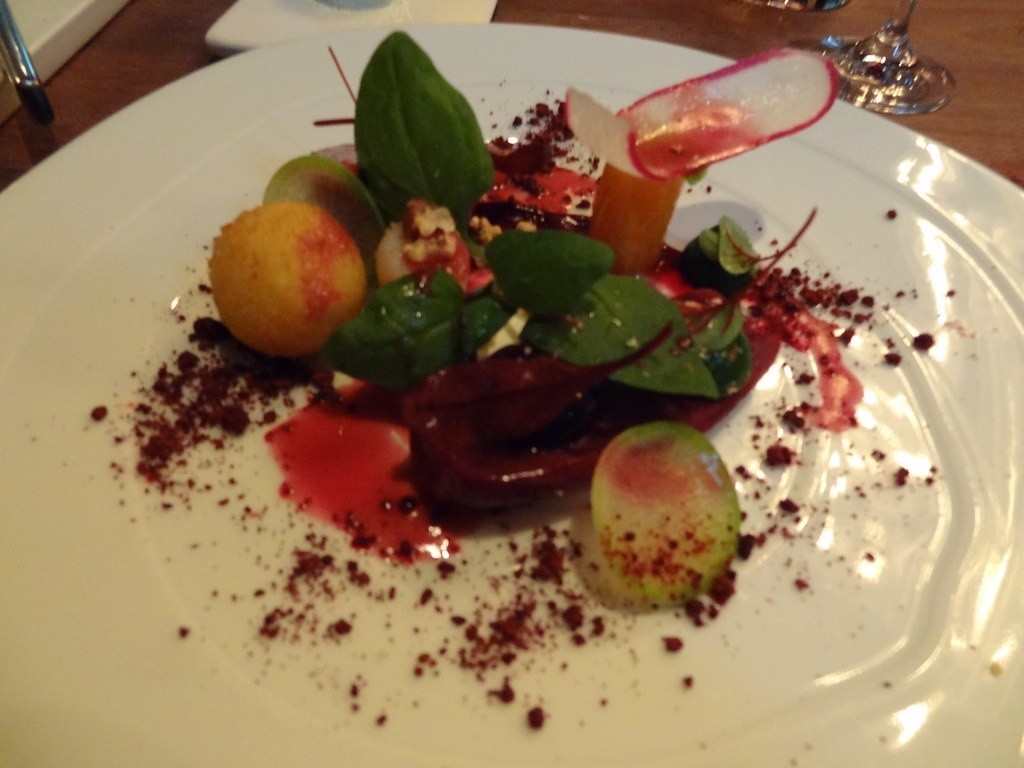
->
[803,0,956,116]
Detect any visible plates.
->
[204,0,499,56]
[0,21,1024,768]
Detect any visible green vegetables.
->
[316,27,817,399]
